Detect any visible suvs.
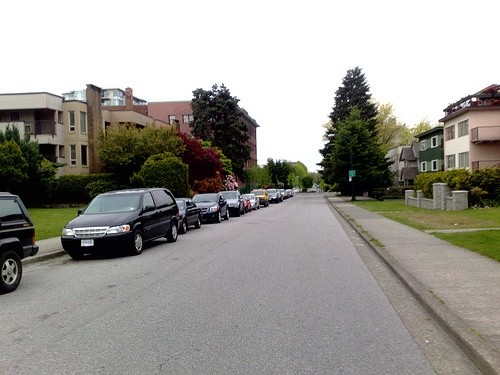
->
[0,191,39,294]
[61,187,180,261]
[218,191,244,217]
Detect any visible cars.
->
[174,198,203,234]
[191,193,229,223]
[242,187,295,214]
